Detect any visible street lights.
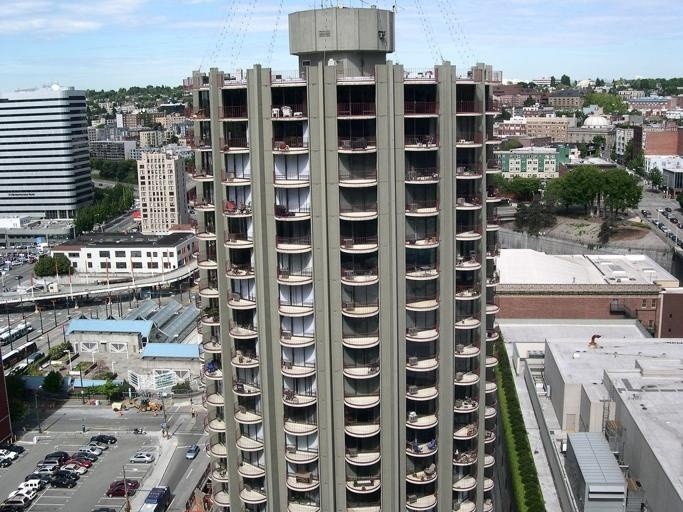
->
[112,362,116,375]
[92,352,96,364]
[94,222,103,233]
[63,350,72,371]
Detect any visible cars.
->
[0,444,24,467]
[79,446,102,456]
[130,454,155,463]
[0,254,37,274]
[186,445,200,459]
[86,441,109,450]
[642,207,683,247]
[107,477,138,499]
[32,451,98,489]
[16,275,24,280]
[0,474,52,512]
[91,435,117,444]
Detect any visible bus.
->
[138,486,170,512]
[0,322,33,346]
[2,341,45,377]
